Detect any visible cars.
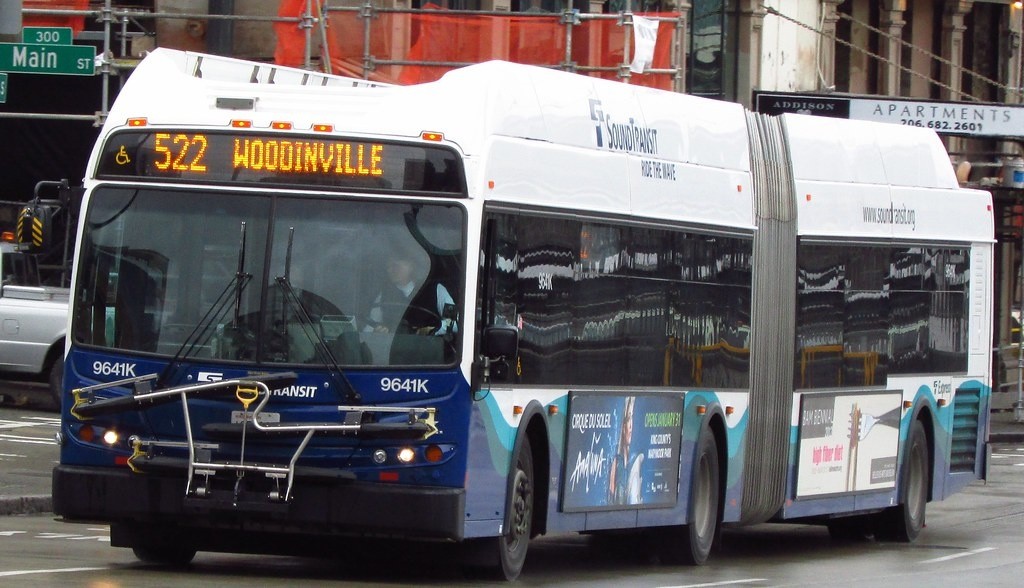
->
[994,309,1024,358]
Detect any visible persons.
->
[365,244,458,341]
[607,395,636,504]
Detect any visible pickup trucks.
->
[1,242,69,411]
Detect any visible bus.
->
[15,47,1000,582]
